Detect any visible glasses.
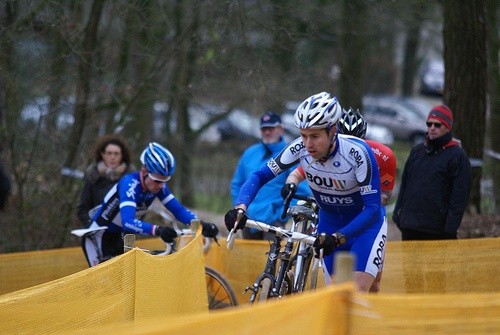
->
[426,121,443,128]
[144,173,173,186]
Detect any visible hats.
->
[427,105,452,130]
[260,112,282,130]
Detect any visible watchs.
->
[335,232,345,247]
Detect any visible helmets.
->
[140,141,175,182]
[293,92,342,130]
[336,106,367,139]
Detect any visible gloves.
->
[224,208,247,234]
[200,220,219,237]
[156,226,178,243]
[313,233,337,258]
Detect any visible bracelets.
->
[152,224,156,236]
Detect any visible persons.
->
[280,106,396,293]
[71,142,218,266]
[392,104,472,293]
[230,113,314,280]
[77,136,131,227]
[225,91,388,294]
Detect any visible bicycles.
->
[151,228,239,310]
[227,183,323,303]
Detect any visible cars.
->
[22,86,443,145]
